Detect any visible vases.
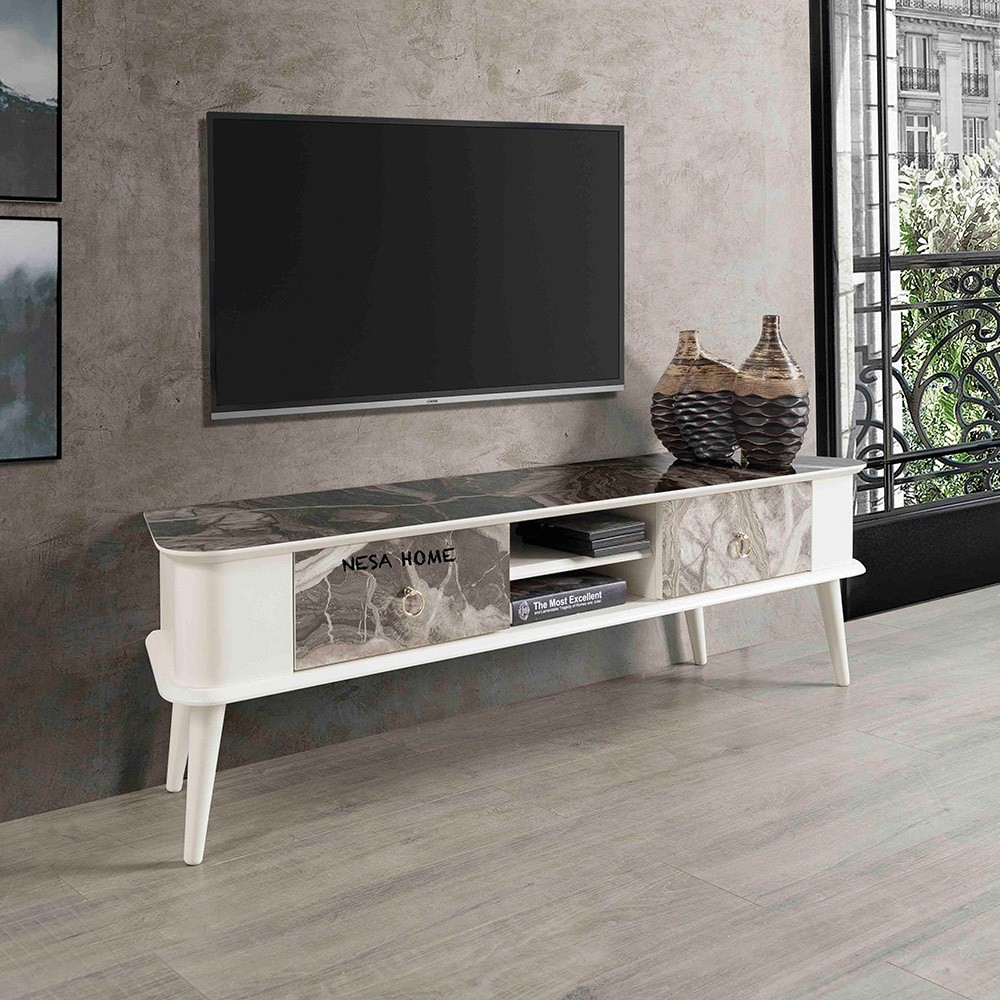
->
[650,329,738,466]
[729,315,809,468]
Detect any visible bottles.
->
[732,315,809,472]
[650,330,741,461]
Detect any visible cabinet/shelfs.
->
[143,453,865,867]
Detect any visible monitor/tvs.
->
[207,111,627,419]
